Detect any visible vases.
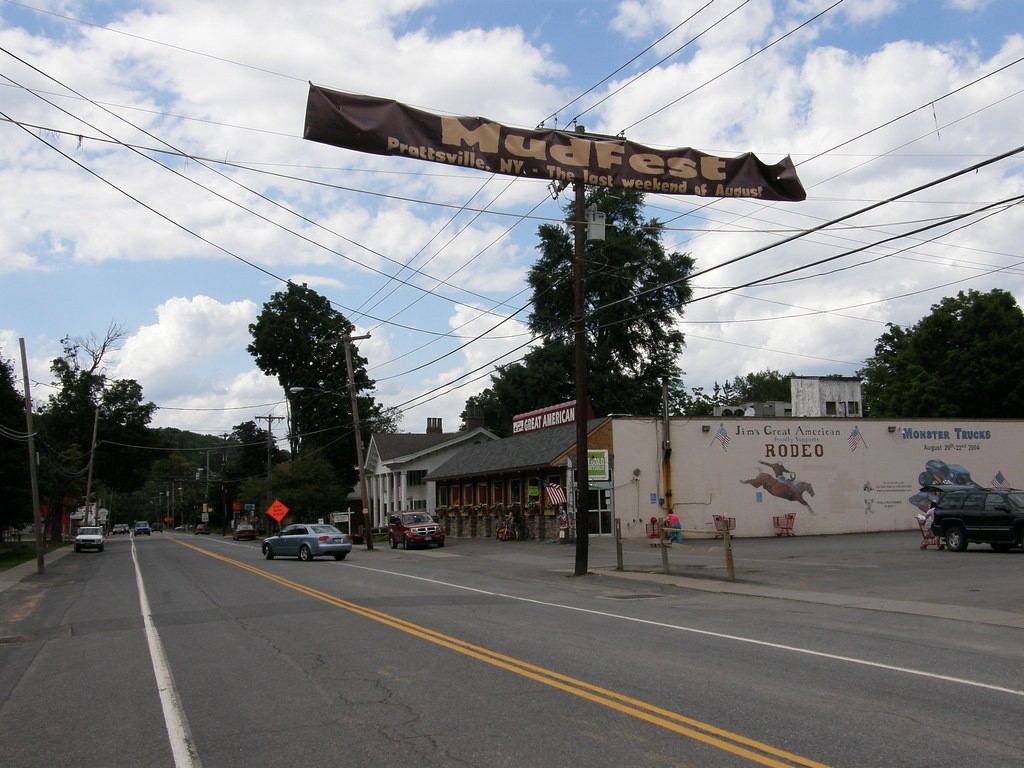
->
[460,512,469,516]
[476,511,487,517]
[524,511,538,516]
[544,509,556,515]
[449,512,458,516]
[490,512,503,516]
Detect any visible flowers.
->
[475,503,487,512]
[461,504,472,513]
[544,502,559,512]
[489,501,503,514]
[447,506,459,513]
[507,499,520,515]
[524,499,539,514]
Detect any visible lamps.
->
[899,426,906,434]
[888,426,896,433]
[702,425,710,432]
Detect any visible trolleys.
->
[773,513,796,537]
[914,516,947,550]
[646,521,669,547]
[712,515,736,539]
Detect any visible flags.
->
[544,482,567,506]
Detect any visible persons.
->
[924,502,945,550]
[663,510,684,544]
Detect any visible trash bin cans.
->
[559,526,569,544]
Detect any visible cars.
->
[232,525,256,541]
[262,524,352,561]
[113,524,130,535]
[175,525,194,531]
[195,524,209,535]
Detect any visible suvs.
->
[134,521,150,536]
[152,523,162,533]
[74,527,104,552]
[919,485,1024,552]
[387,510,445,550]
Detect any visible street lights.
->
[159,489,174,531]
[289,387,373,551]
